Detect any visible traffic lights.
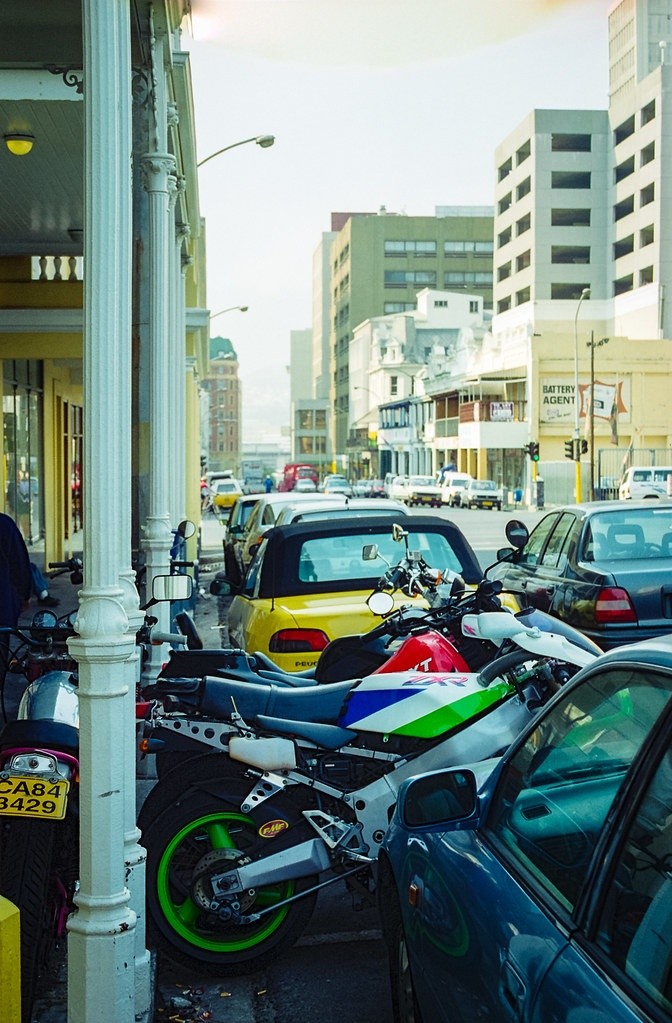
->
[531,442,539,461]
[524,441,532,456]
[564,440,573,460]
[582,439,588,454]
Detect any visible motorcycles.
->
[0,520,639,1023]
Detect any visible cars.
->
[199,453,499,612]
[374,631,671,1023]
[489,498,672,653]
[208,514,523,678]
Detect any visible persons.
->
[8,470,29,539]
[201,477,209,509]
[0,513,34,724]
[30,562,60,607]
[264,476,273,493]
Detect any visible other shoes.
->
[73,526,78,532]
[80,524,83,529]
[39,594,60,607]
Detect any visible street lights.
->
[573,286,592,503]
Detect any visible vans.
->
[617,465,672,503]
